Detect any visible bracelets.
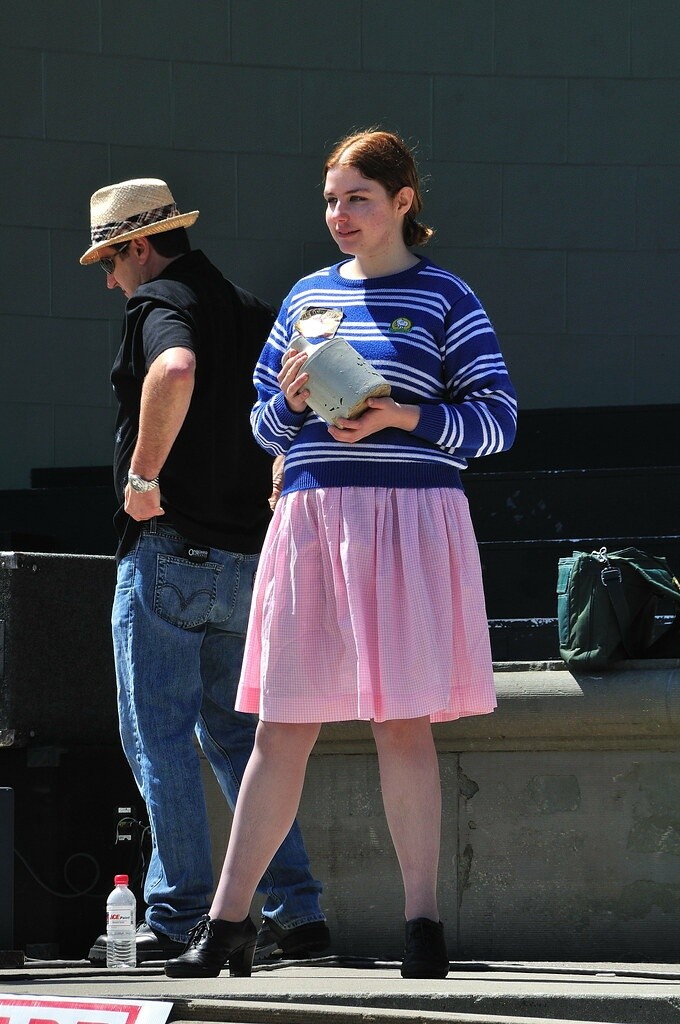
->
[273,483,282,491]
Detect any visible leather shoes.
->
[253,911,331,965]
[87,918,193,965]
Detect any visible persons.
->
[164,131,517,980]
[79,177,331,965]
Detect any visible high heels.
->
[164,911,257,978]
[400,916,451,980]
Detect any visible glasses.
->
[98,239,131,275]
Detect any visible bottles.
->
[106,874,136,969]
[282,335,391,431]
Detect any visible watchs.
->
[128,469,159,493]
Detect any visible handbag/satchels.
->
[556,546,680,674]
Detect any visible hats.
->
[79,178,198,267]
